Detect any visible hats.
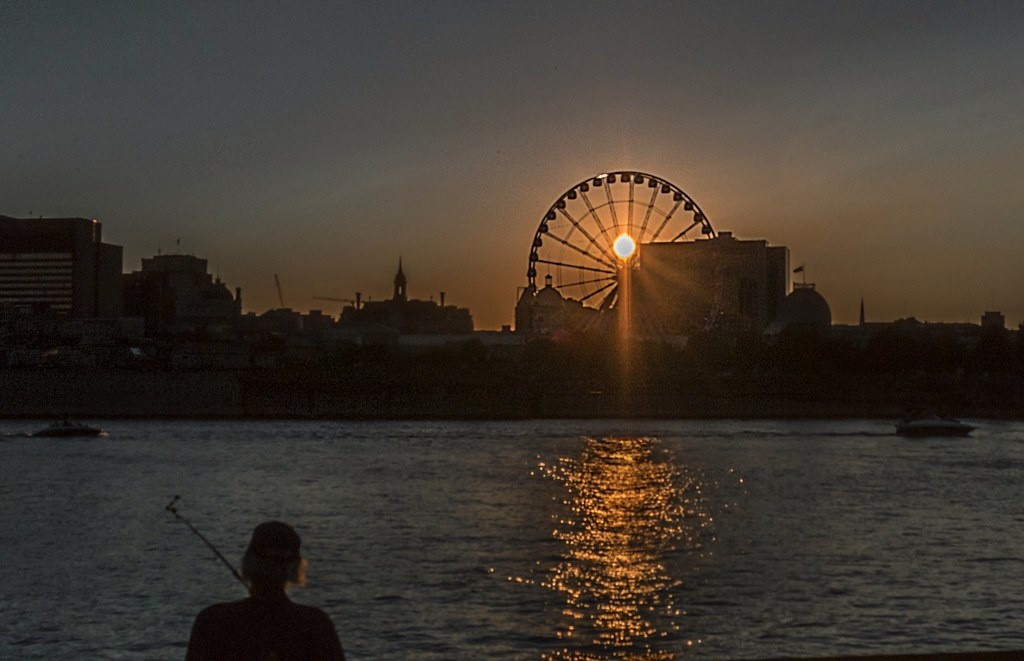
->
[247,520,301,562]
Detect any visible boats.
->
[892,406,977,436]
[33,420,102,437]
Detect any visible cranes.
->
[312,291,367,310]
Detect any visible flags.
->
[793,266,804,272]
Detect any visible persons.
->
[185,520,347,661]
